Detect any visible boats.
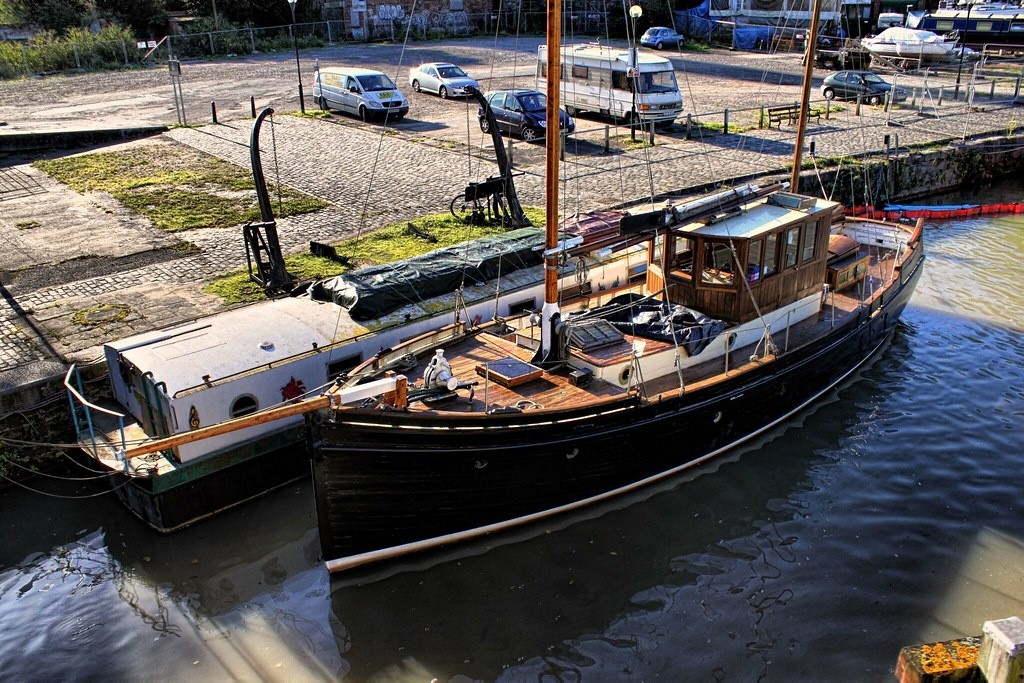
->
[861,27,960,62]
[61,177,790,536]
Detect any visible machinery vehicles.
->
[813,37,904,72]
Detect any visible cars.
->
[409,62,479,98]
[479,90,575,142]
[820,69,907,105]
[641,26,685,49]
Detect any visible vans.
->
[536,44,683,129]
[313,66,409,122]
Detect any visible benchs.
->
[768,104,820,130]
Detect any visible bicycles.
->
[451,175,512,221]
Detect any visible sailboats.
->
[127,1,924,582]
